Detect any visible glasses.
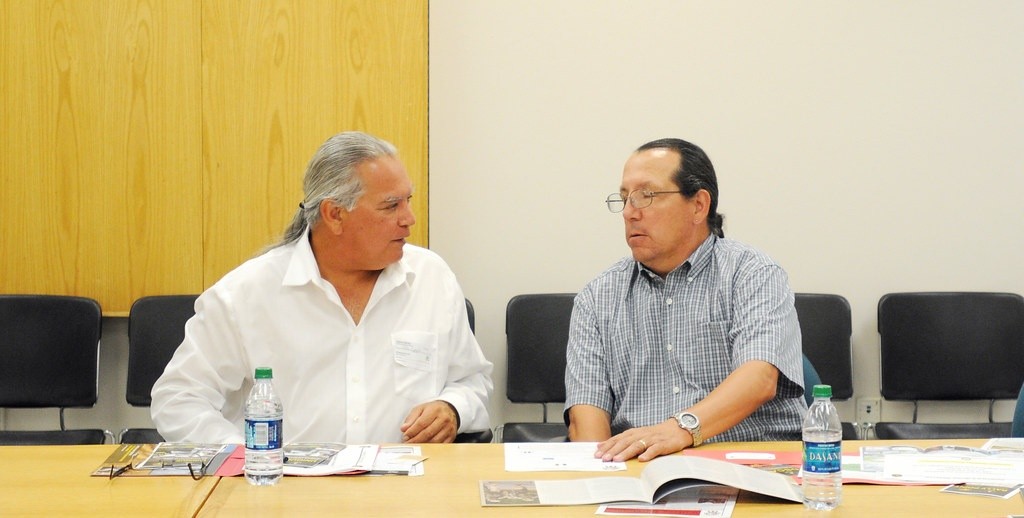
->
[605,188,680,213]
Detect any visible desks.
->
[0,438,1024,518]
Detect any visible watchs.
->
[669,411,703,447]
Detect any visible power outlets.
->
[856,398,882,429]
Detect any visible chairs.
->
[451,299,493,443]
[498,292,577,441]
[0,293,117,446]
[795,292,860,442]
[121,293,199,446]
[865,291,1024,440]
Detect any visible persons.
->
[150,133,495,446]
[562,137,813,462]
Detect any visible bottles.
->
[802,385,842,511]
[244,368,284,485]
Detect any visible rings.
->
[639,440,647,450]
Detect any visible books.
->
[478,455,806,506]
